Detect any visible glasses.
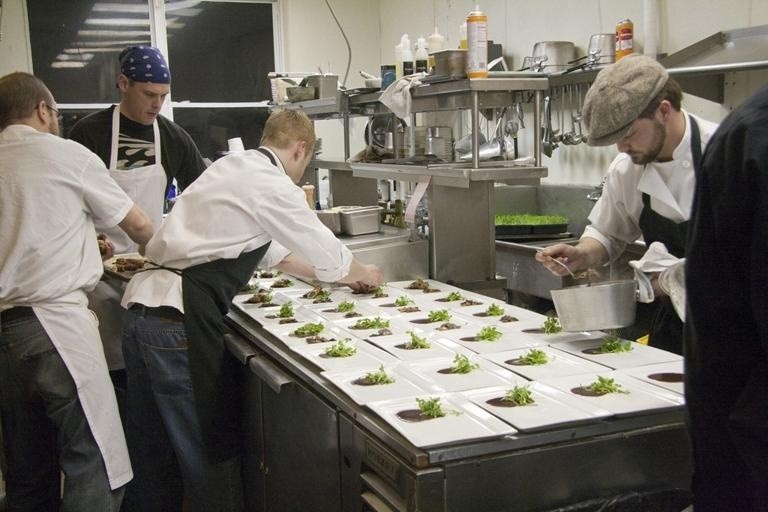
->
[35,102,61,118]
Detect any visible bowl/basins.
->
[364,113,408,149]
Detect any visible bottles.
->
[380,26,445,92]
[614,17,635,64]
[459,3,488,81]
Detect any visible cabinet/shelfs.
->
[222,333,342,511]
[269,76,550,282]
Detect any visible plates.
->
[102,252,147,280]
[231,269,685,450]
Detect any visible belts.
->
[133,303,186,322]
[0,305,33,324]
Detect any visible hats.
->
[580,53,670,146]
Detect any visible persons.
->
[531,50,721,357]
[682,82,767,511]
[0,68,153,511]
[117,104,392,511]
[61,43,209,434]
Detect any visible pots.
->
[548,279,640,333]
[516,33,615,77]
